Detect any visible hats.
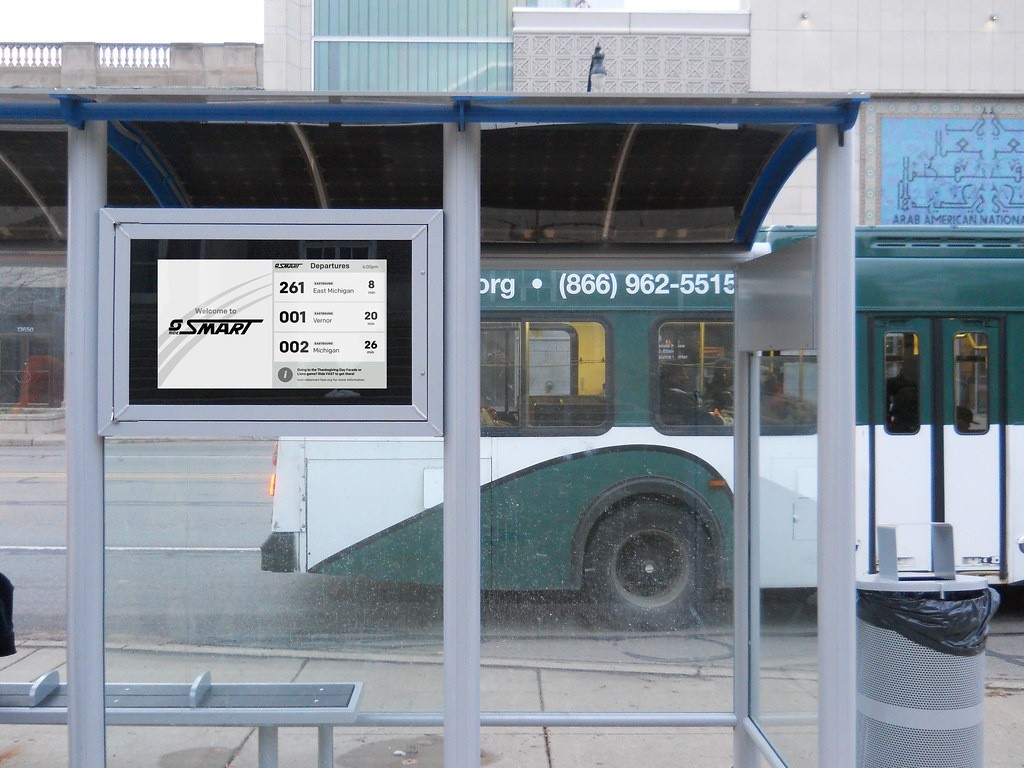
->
[958,405,980,425]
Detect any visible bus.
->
[257,225,1024,641]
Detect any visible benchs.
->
[0,670,364,768]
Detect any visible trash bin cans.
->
[857,521,993,767]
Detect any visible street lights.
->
[585,42,608,93]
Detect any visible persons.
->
[760,364,795,424]
[891,357,918,433]
[956,406,980,430]
[702,359,733,412]
[659,359,724,425]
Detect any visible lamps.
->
[800,13,808,19]
[990,15,997,20]
